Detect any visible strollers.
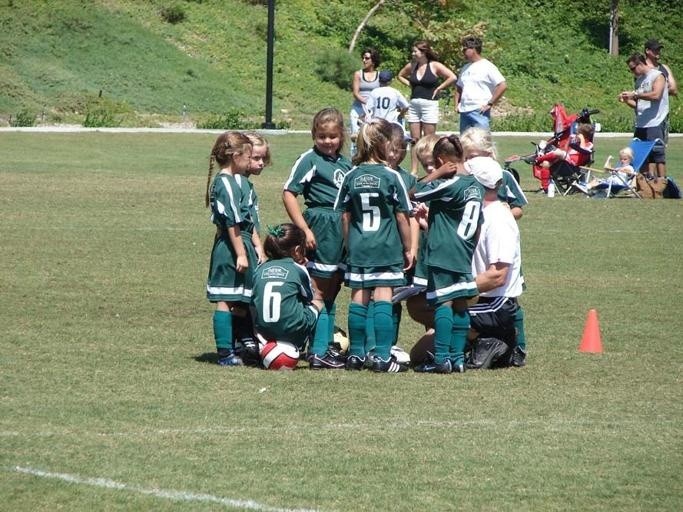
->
[532,106,600,192]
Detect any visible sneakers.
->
[413,335,527,372]
[305,343,408,373]
[207,332,261,367]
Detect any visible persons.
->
[364,69,411,122]
[205,106,529,374]
[634,38,676,147]
[576,146,634,196]
[396,41,457,177]
[453,36,508,137]
[537,124,593,196]
[617,51,670,180]
[351,46,380,157]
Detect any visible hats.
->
[644,39,665,52]
[619,146,635,160]
[462,155,504,191]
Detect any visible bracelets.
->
[487,99,493,106]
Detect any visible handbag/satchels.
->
[634,172,681,199]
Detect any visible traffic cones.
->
[577,308,604,353]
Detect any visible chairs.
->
[549,117,668,198]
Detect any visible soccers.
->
[331,327,349,353]
[260,340,299,369]
